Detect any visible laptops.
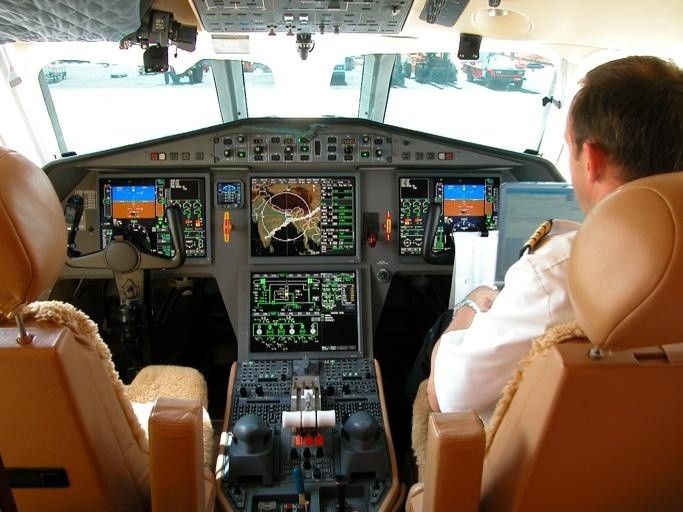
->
[494,182,588,293]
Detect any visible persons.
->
[404,55,683,488]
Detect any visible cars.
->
[42,56,545,92]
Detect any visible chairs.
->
[0,149,217,510]
[404,173,680,512]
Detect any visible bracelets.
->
[452,298,481,319]
